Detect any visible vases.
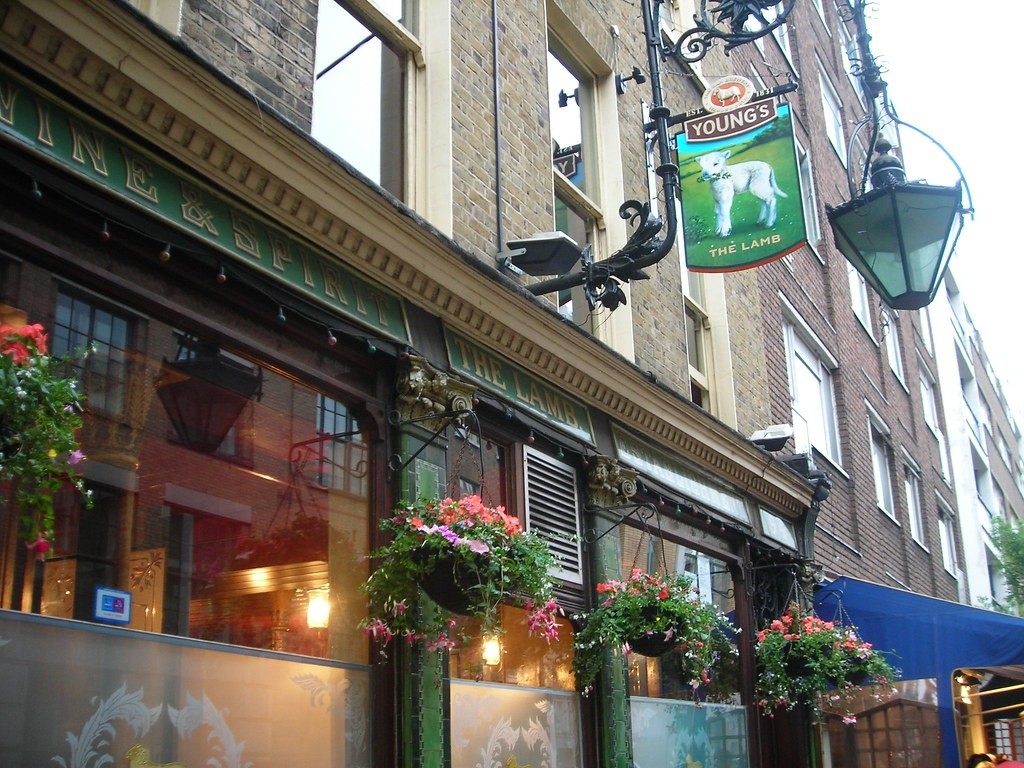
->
[627,605,685,656]
[412,547,523,614]
[782,642,832,676]
[0,413,22,458]
[844,658,870,686]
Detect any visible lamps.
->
[307,590,329,640]
[153,335,262,452]
[483,637,502,671]
[496,230,584,276]
[527,1,977,310]
[954,670,981,687]
[749,424,796,452]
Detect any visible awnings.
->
[660,609,739,702]
[811,569,1024,768]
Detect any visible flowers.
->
[567,570,743,709]
[354,497,566,654]
[0,324,99,558]
[752,602,903,726]
